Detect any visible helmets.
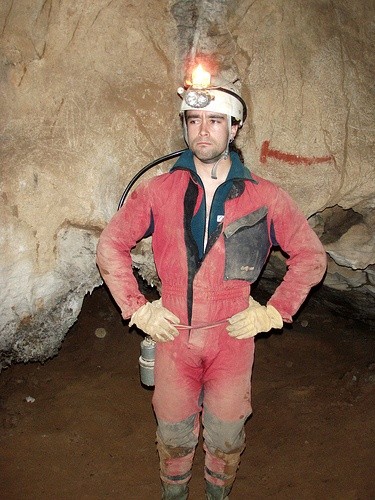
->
[178,75,247,129]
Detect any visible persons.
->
[95,77,327,500]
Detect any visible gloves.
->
[128,296,180,343]
[226,295,284,340]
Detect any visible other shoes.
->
[205,480,232,500]
[161,480,189,500]
